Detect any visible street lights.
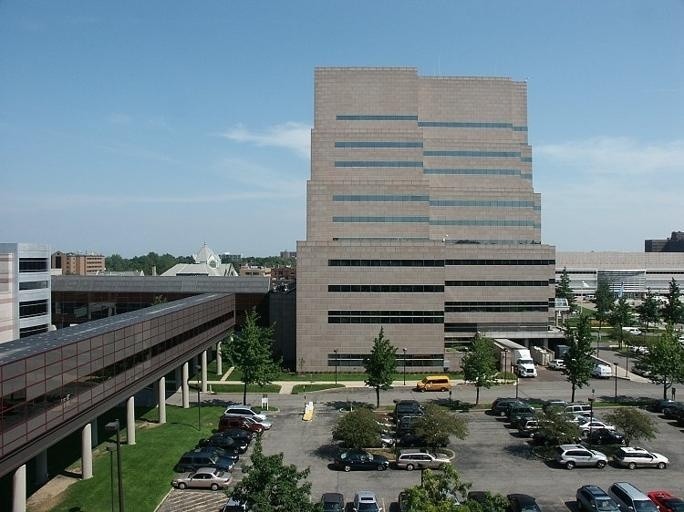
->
[510,361,518,399]
[105,444,117,512]
[332,347,338,384]
[104,417,124,511]
[613,362,619,402]
[194,365,202,432]
[596,328,600,357]
[403,345,408,386]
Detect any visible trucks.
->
[493,337,538,378]
[591,363,613,380]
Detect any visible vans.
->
[415,375,450,393]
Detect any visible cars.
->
[171,404,273,491]
[547,358,567,370]
[85,375,116,388]
[320,490,345,511]
[221,495,251,511]
[583,295,596,301]
[353,490,382,511]
[677,337,684,346]
[0,394,75,415]
[629,328,642,335]
[575,481,684,512]
[631,343,657,356]
[334,398,450,473]
[489,394,669,471]
[398,490,541,511]
